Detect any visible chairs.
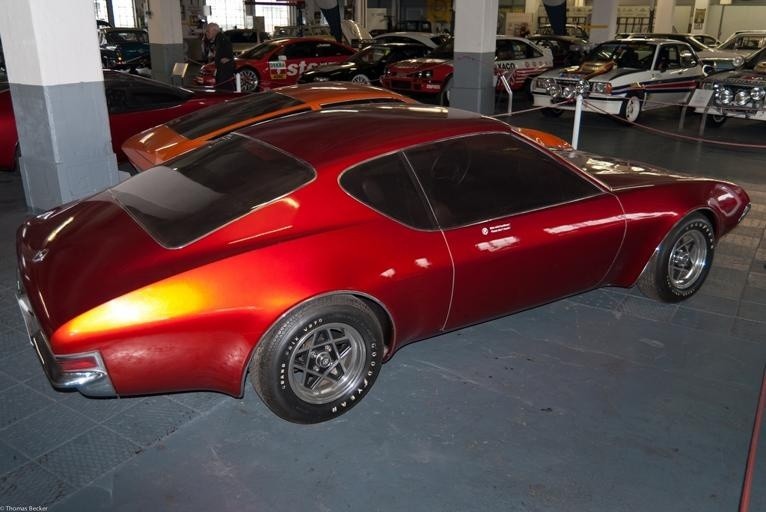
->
[619,48,638,68]
[383,149,456,231]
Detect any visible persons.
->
[206,23,235,91]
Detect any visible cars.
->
[0,12,765,186]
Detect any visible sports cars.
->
[10,97,755,433]
